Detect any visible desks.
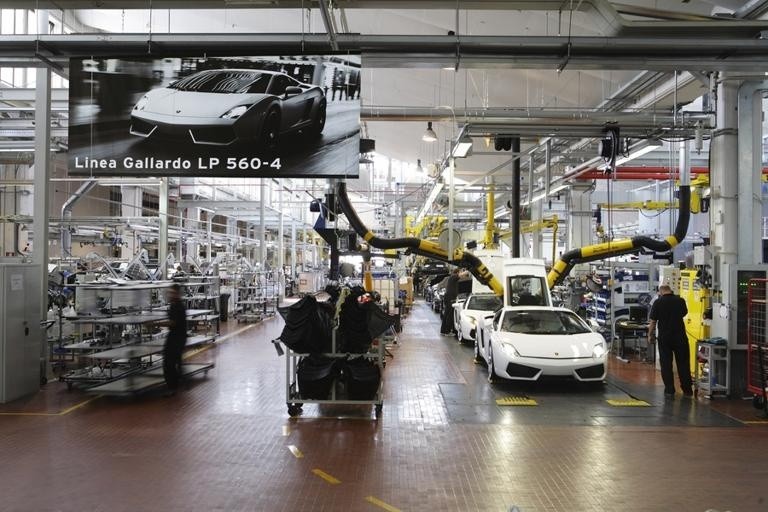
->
[616,320,656,363]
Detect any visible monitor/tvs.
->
[629,306,647,324]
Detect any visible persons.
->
[515,278,537,305]
[659,395,693,423]
[438,267,471,337]
[188,264,199,273]
[647,283,694,399]
[411,270,421,293]
[174,266,186,273]
[150,285,188,398]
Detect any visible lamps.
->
[413,140,424,178]
[421,107,437,142]
[451,134,473,158]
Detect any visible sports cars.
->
[130,68,326,165]
[452,250,509,344]
[474,258,608,390]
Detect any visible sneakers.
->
[664,387,675,394]
[683,390,693,397]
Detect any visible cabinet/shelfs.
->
[694,342,731,397]
[54,265,333,401]
[586,261,655,353]
[270,266,415,425]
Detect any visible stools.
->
[618,334,642,362]
